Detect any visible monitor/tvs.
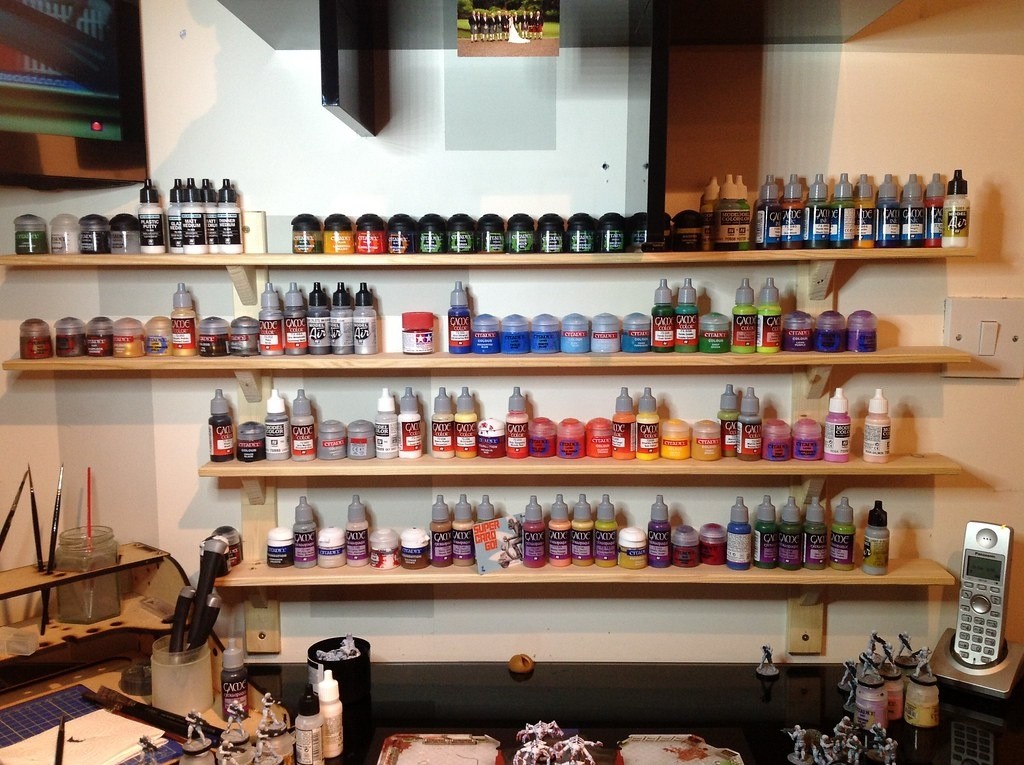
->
[0,0,150,183]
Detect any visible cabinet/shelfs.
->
[0,211,975,694]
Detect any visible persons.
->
[137,734,160,765]
[218,740,239,765]
[224,700,244,738]
[786,630,933,765]
[468,10,544,43]
[758,642,777,671]
[258,692,282,729]
[253,724,278,763]
[185,709,208,746]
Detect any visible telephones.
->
[925,521,1024,700]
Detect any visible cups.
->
[150,632,213,718]
[50,525,121,625]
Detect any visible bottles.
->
[220,638,343,765]
[650,278,699,353]
[506,389,529,459]
[138,179,166,254]
[522,494,618,568]
[698,173,947,252]
[168,178,244,254]
[612,387,659,461]
[941,168,971,247]
[822,387,891,463]
[259,282,378,355]
[291,495,495,568]
[375,387,477,459]
[208,389,234,462]
[717,384,761,461]
[448,281,471,354]
[648,494,890,575]
[264,389,316,462]
[171,283,198,357]
[731,278,781,353]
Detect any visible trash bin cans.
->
[306,635,373,755]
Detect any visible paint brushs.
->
[54,712,66,765]
[78,684,226,749]
[0,463,65,634]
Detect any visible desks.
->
[245,662,1024,765]
